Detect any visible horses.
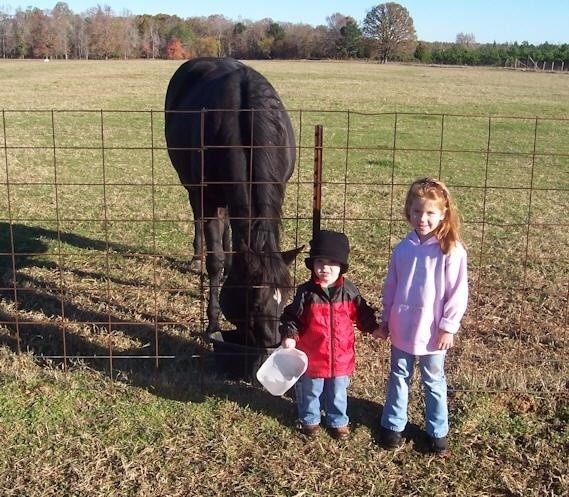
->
[163,57,305,387]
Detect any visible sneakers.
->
[381,426,401,449]
[331,427,350,440]
[302,424,320,436]
[427,434,448,452]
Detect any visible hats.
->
[256,343,308,397]
[304,230,350,274]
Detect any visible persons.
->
[275,227,388,441]
[372,176,470,457]
